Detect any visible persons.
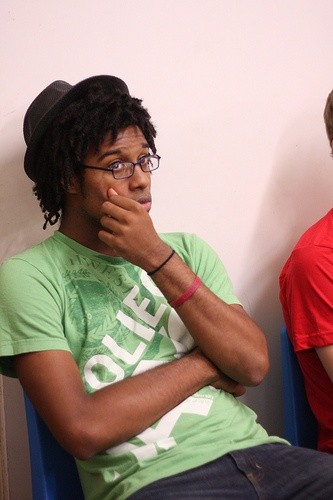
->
[1,75,333,500]
[280,89,333,455]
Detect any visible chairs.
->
[280,324,319,449]
[23,389,85,500]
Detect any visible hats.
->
[22,74,129,182]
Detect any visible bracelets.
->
[147,251,175,275]
[168,276,202,307]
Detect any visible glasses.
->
[81,154,161,180]
